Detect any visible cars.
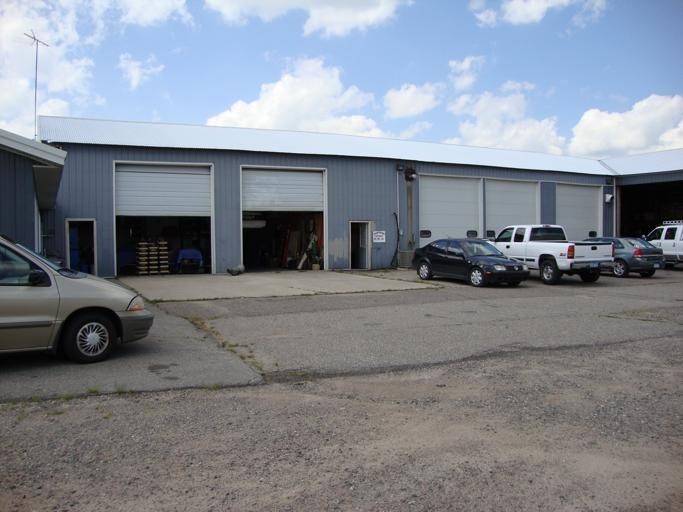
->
[410,235,530,289]
[579,235,663,279]
[0,234,155,366]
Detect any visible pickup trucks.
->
[640,219,683,267]
[481,222,618,286]
